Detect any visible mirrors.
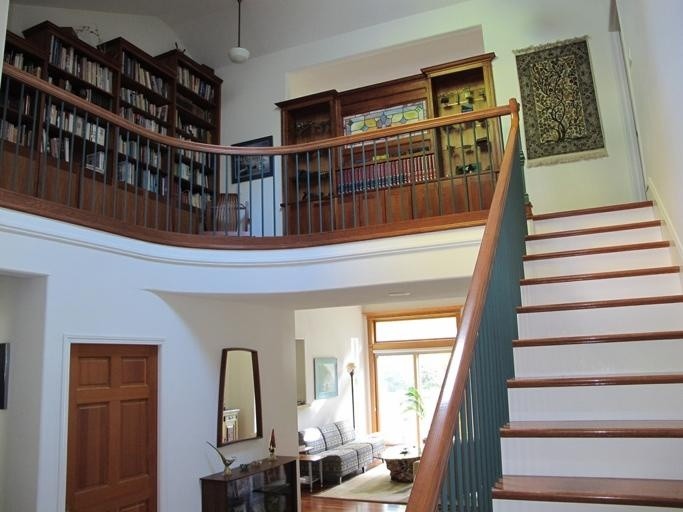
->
[216,348,264,448]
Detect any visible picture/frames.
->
[314,357,339,400]
[229,135,275,184]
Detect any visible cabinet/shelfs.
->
[200,455,299,512]
[222,408,240,444]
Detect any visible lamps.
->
[345,362,358,430]
[227,1,250,64]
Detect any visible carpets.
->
[312,462,498,508]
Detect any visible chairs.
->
[298,419,384,484]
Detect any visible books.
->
[0,33,215,212]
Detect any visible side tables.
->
[299,453,328,493]
